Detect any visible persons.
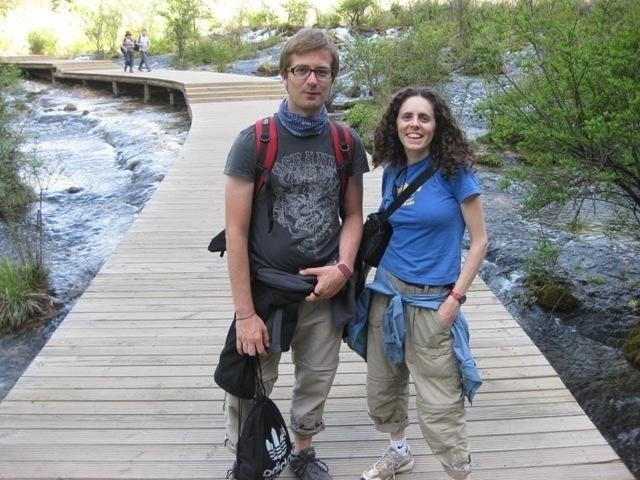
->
[362,87,488,479]
[121,31,135,72]
[137,28,152,72]
[207,28,369,479]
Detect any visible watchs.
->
[450,290,466,304]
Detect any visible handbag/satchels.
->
[361,212,393,268]
[134,41,141,51]
[232,395,291,479]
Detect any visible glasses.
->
[286,64,333,79]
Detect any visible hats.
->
[125,31,132,36]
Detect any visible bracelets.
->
[235,312,256,319]
[338,263,352,279]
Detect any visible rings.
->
[313,291,319,297]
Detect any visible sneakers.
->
[360,444,414,480]
[287,446,333,480]
